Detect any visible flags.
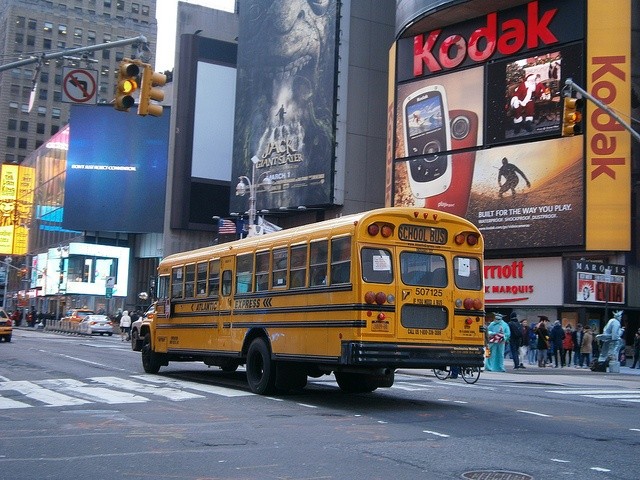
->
[219,219,237,235]
[258,216,283,234]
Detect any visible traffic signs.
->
[106,288,112,299]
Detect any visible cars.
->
[78,314,113,336]
[60,314,73,327]
[0,309,12,342]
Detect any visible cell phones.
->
[402,86,452,199]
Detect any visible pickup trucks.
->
[69,310,96,332]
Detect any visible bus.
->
[141,207,487,395]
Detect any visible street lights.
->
[236,156,272,238]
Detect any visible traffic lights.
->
[20,269,26,280]
[137,65,167,118]
[114,57,139,112]
[59,272,63,283]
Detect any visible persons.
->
[549,320,565,368]
[528,323,537,365]
[596,310,626,364]
[518,319,530,368]
[413,113,422,124]
[504,341,514,359]
[574,322,584,366]
[485,312,511,372]
[630,328,640,369]
[498,157,531,199]
[12,305,62,327]
[511,74,537,134]
[534,316,550,367]
[580,326,593,367]
[591,333,599,358]
[105,309,144,341]
[450,365,460,378]
[276,104,287,126]
[562,322,575,367]
[547,323,555,363]
[508,311,523,369]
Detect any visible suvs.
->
[131,302,158,351]
